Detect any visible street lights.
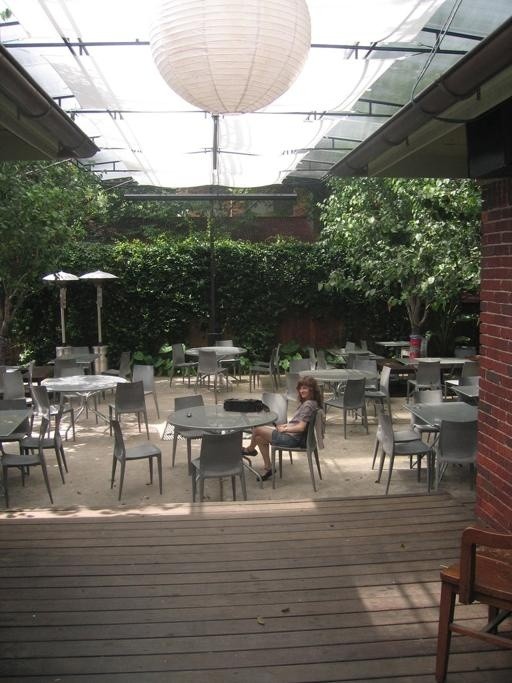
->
[41,268,81,348]
[81,270,119,372]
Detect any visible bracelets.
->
[285,426,288,432]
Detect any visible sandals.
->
[256,469,276,481]
[243,448,258,456]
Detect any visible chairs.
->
[435,526,512,683]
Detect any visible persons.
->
[242,377,322,481]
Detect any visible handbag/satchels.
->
[224,399,263,412]
